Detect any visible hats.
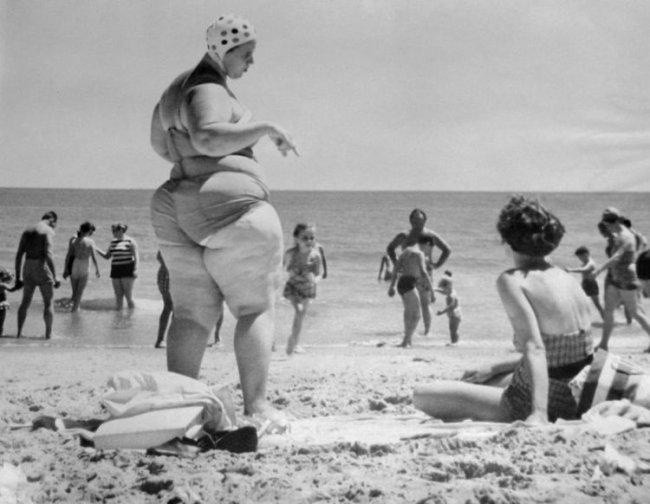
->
[204,14,256,74]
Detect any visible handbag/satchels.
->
[93,368,240,455]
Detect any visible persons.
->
[0,211,61,339]
[63,222,100,311]
[215,315,223,343]
[409,193,595,429]
[155,250,173,347]
[283,223,327,354]
[149,13,304,427]
[379,208,451,345]
[567,208,650,353]
[95,222,138,309]
[434,271,462,343]
